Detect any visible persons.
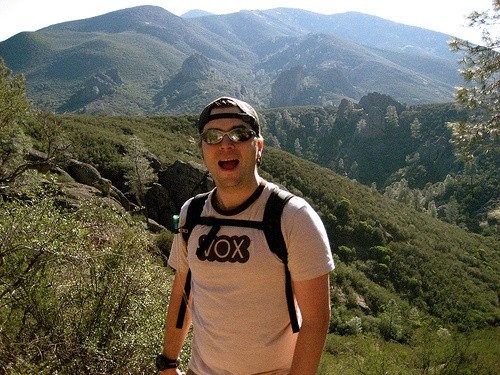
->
[161,97,338,375]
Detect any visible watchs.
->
[154,354,180,371]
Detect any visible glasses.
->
[200,128,258,145]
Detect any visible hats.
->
[198,96,259,136]
